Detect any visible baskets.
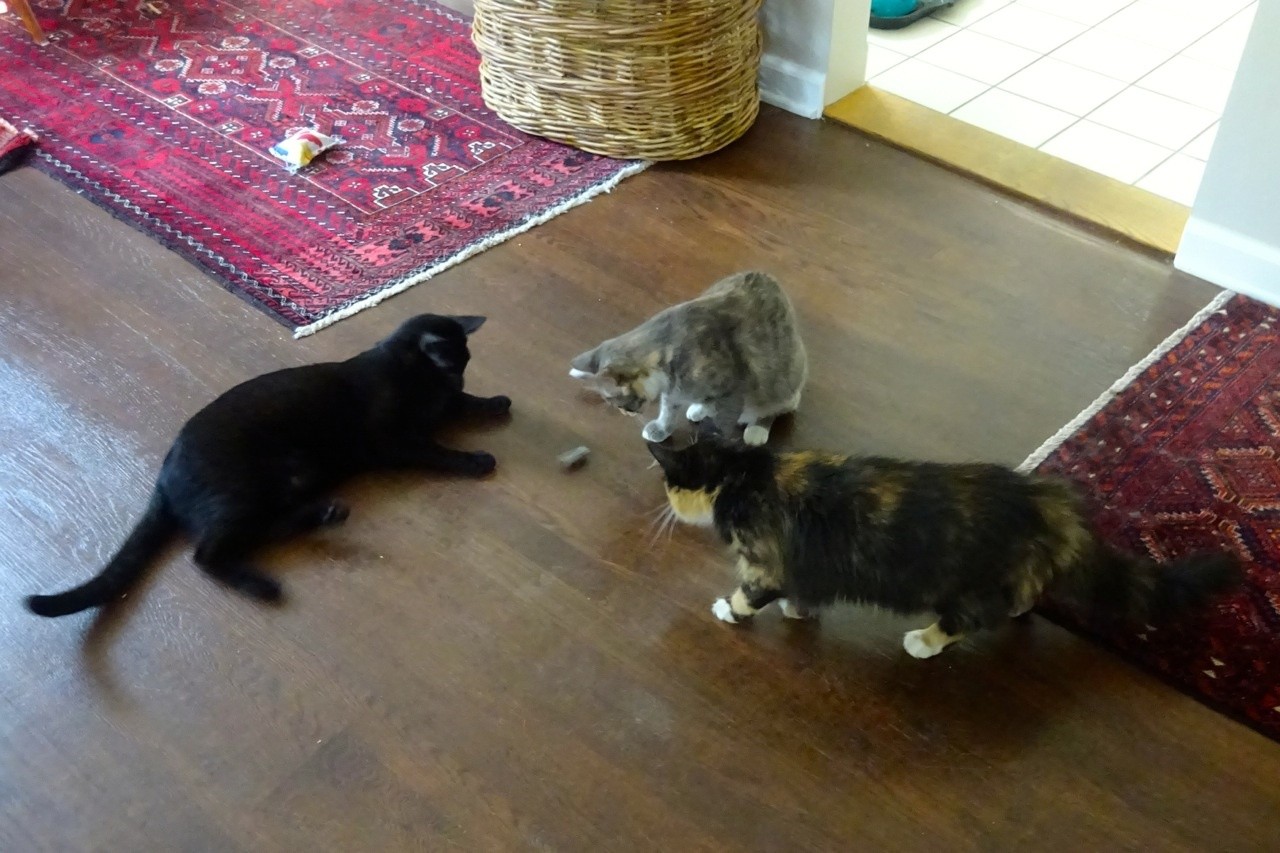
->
[470,0,765,160]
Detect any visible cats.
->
[21,311,513,618]
[647,415,1098,660]
[569,270,810,447]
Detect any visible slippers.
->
[869,0,949,30]
